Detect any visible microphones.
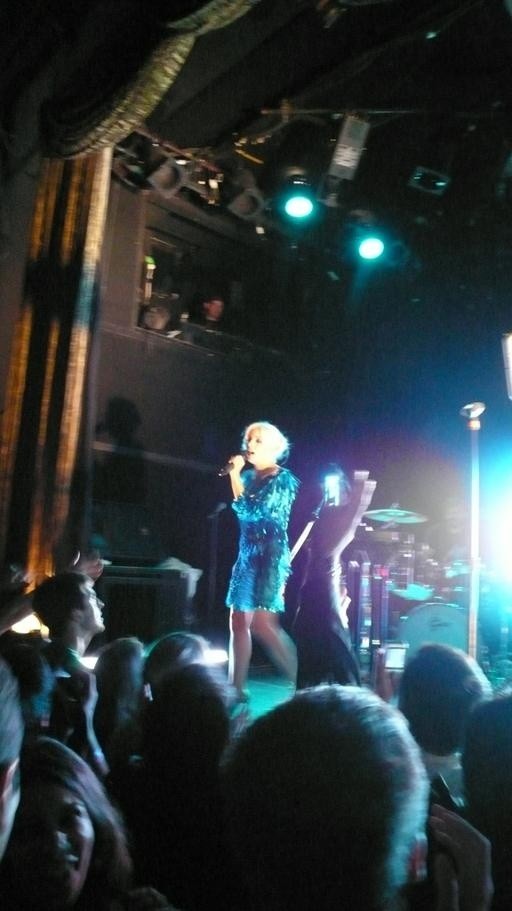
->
[217,448,251,481]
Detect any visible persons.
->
[192,292,225,352]
[220,420,298,705]
